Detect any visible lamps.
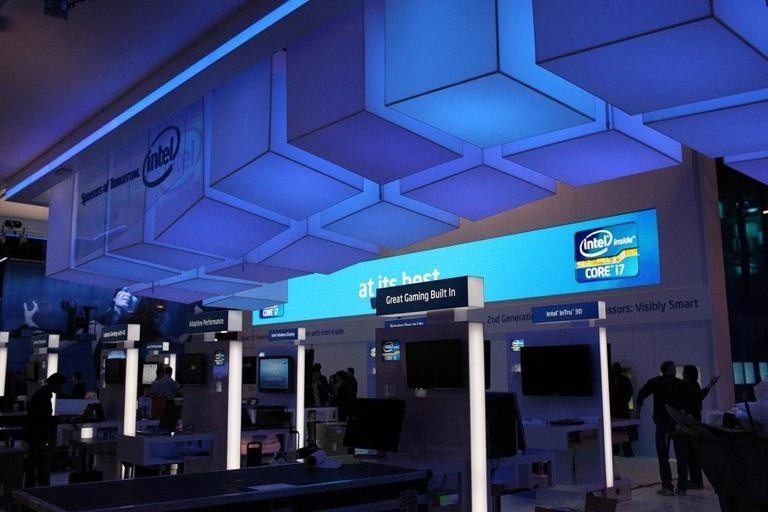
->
[151,298,166,312]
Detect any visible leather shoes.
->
[657,479,704,495]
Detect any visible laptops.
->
[71,403,105,422]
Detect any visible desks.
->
[0,401,641,511]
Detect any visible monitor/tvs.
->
[24,360,38,381]
[176,353,209,384]
[520,343,594,398]
[159,399,183,433]
[142,362,160,384]
[405,339,491,389]
[257,355,294,393]
[105,358,126,384]
[342,397,406,453]
[660,364,702,390]
[485,391,527,455]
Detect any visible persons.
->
[337,367,358,421]
[311,361,330,407]
[157,367,178,431]
[24,373,68,491]
[681,363,723,491]
[633,360,690,497]
[608,360,636,459]
